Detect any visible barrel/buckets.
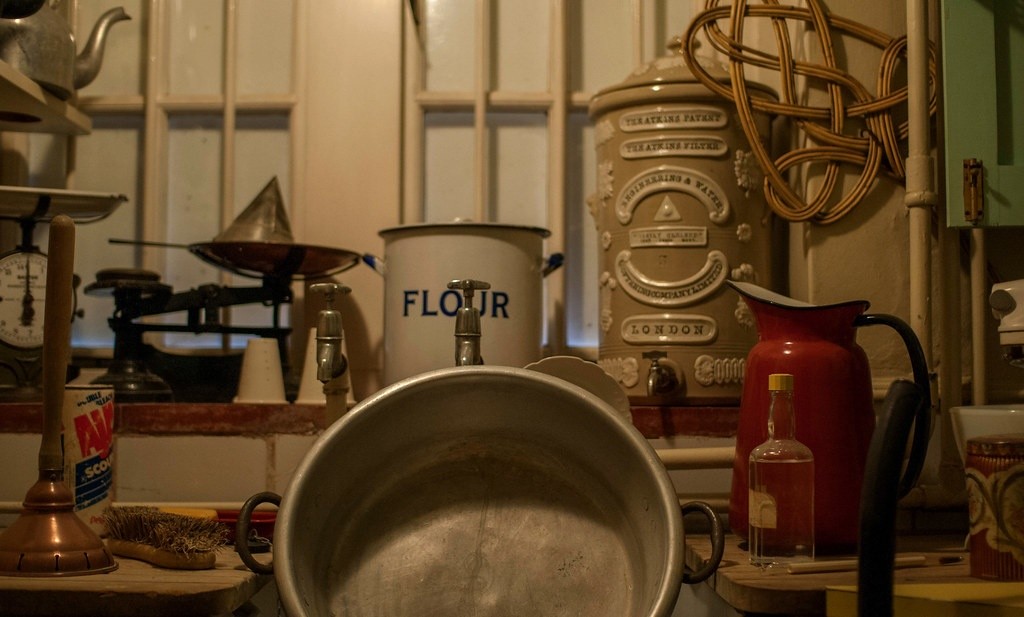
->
[590,35,784,403]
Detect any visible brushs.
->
[96,503,233,571]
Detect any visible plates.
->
[523,355,634,423]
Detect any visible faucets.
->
[307,281,351,382]
[446,279,493,376]
[641,350,667,398]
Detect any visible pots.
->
[236,365,722,617]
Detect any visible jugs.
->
[723,275,932,552]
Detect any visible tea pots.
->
[1,0,132,189]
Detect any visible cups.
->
[361,223,567,398]
[233,337,290,403]
[964,435,1024,584]
[294,327,355,402]
[950,404,1024,463]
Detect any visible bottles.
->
[63,383,116,537]
[747,374,818,572]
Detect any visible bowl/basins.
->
[212,509,276,544]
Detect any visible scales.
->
[0,183,127,388]
